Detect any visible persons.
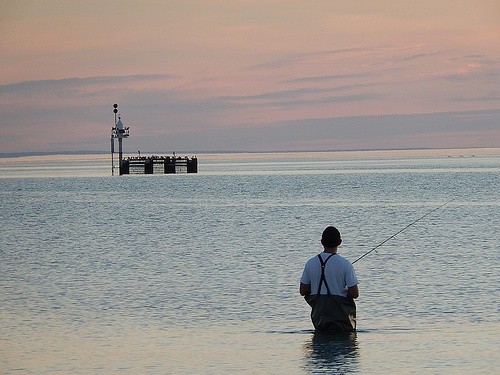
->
[300,226,359,333]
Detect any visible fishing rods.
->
[351,194,461,266]
